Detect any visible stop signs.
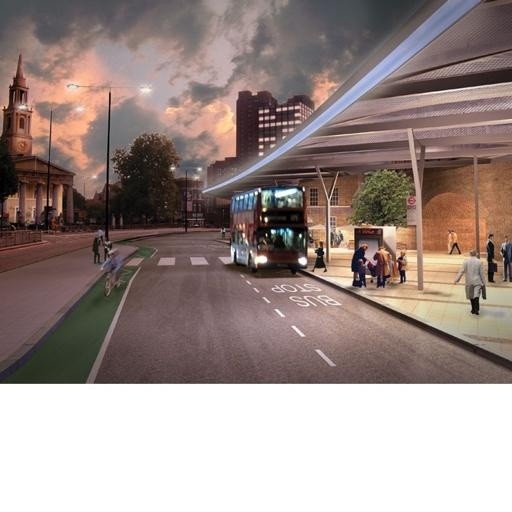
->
[407,196,416,205]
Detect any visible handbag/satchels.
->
[493,263,497,272]
[482,286,486,299]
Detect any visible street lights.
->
[16,103,85,229]
[66,83,152,238]
[170,166,203,233]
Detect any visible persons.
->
[35,209,66,233]
[503,237,512,282]
[498,235,509,282]
[449,229,460,255]
[100,249,124,287]
[446,230,452,252]
[92,237,101,264]
[486,234,495,283]
[96,228,106,246]
[309,240,328,273]
[453,248,486,316]
[102,236,111,261]
[350,243,409,288]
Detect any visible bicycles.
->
[99,246,121,296]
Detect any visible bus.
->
[230,186,309,274]
